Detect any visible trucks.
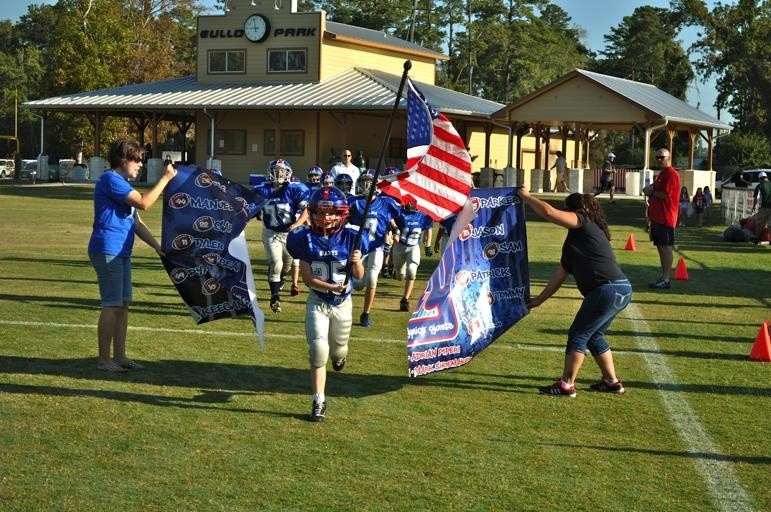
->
[49,159,89,181]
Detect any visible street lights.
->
[467,65,474,95]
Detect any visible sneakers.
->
[312,400,327,421]
[360,313,369,326]
[270,277,299,312]
[590,377,624,394]
[537,379,576,398]
[400,297,409,311]
[656,277,671,289]
[382,264,393,278]
[333,358,345,371]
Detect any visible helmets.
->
[266,159,292,185]
[307,166,419,234]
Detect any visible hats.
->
[758,172,768,179]
[608,153,616,157]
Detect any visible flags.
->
[375,76,472,236]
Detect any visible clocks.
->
[243,14,271,43]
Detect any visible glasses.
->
[344,155,350,157]
[656,156,669,159]
[128,155,143,163]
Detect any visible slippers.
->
[96,360,144,373]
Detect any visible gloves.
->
[425,247,433,256]
[384,243,391,256]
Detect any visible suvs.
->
[716,168,771,198]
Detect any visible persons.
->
[731,164,752,187]
[643,148,680,289]
[434,215,456,253]
[87,141,178,373]
[254,159,433,327]
[550,151,569,192]
[750,171,771,245]
[286,186,369,422]
[678,186,713,227]
[329,149,361,195]
[516,187,632,397]
[164,155,172,165]
[645,196,652,232]
[592,152,617,204]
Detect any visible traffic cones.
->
[750,320,771,362]
[674,257,689,280]
[625,234,637,250]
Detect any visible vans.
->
[20,160,38,177]
[0,158,15,178]
